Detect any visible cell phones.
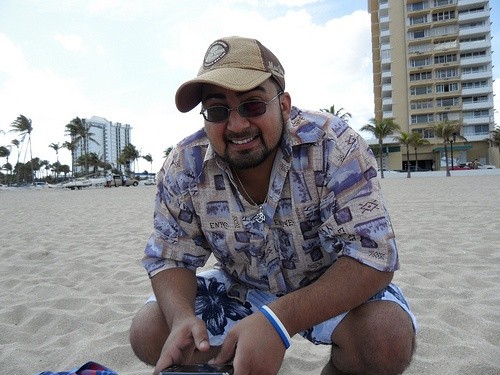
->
[160,364,234,375]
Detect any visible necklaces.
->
[231,166,268,224]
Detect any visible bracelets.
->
[260,305,294,349]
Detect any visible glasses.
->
[200,92,283,123]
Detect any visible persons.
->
[122,175,127,185]
[129,35,416,375]
[106,174,112,187]
[472,158,479,169]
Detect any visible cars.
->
[104,175,139,187]
[458,162,495,170]
[431,159,458,171]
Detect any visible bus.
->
[133,173,157,185]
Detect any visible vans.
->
[35,182,46,189]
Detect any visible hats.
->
[175,36,285,113]
